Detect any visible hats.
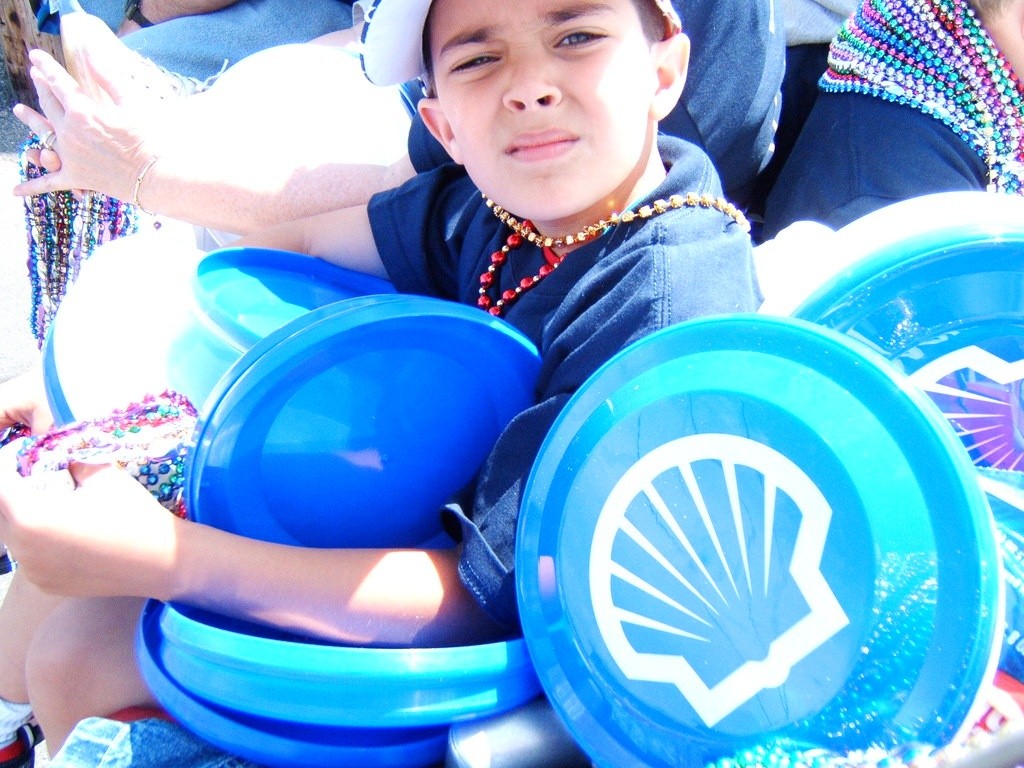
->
[359,0,433,85]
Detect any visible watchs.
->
[125,0,154,28]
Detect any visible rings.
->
[41,130,52,151]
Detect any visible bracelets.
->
[134,155,157,217]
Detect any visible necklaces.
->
[480,192,752,247]
[20,131,138,353]
[816,0,1023,197]
[5,389,202,529]
[477,219,613,317]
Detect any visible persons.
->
[761,0,1024,241]
[771,0,859,172]
[38,0,354,86]
[1,1,759,767]
[15,0,787,238]
